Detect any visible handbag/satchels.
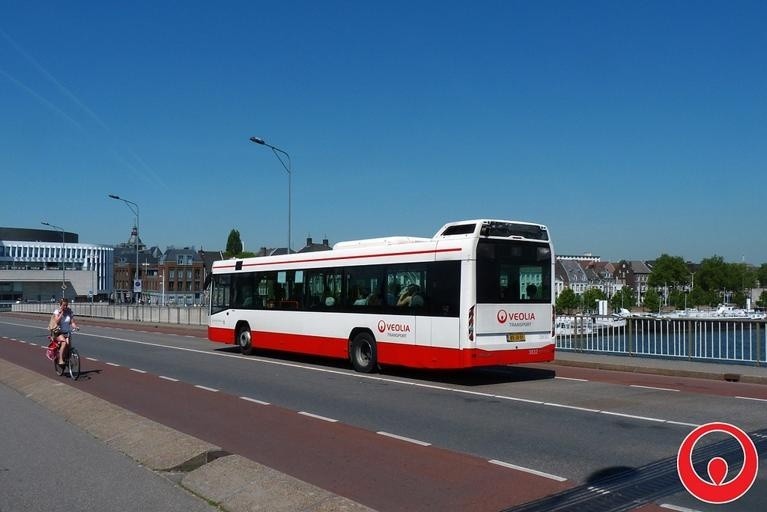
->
[47,314,62,334]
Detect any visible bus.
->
[208,219,556,374]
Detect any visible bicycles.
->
[51,328,80,380]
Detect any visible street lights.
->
[249,136,291,254]
[108,194,139,307]
[41,221,64,301]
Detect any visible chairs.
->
[241,286,423,310]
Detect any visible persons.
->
[526,285,537,299]
[352,287,368,306]
[46,298,79,368]
[396,286,417,307]
